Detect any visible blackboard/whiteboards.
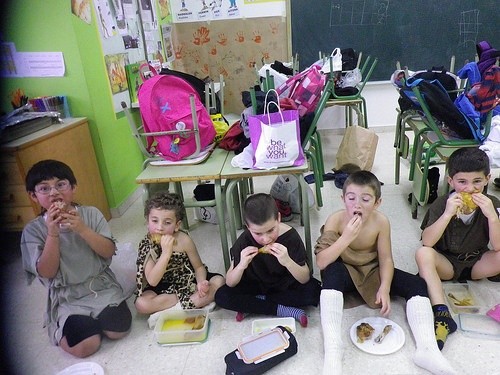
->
[286,0,500,86]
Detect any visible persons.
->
[134,192,226,329]
[313,170,457,375]
[414,147,500,352]
[20,159,132,358]
[214,193,322,328]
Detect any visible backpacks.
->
[139,63,325,170]
[391,41,500,145]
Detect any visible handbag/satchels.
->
[259,47,363,97]
[331,118,379,173]
[270,174,315,213]
[193,196,230,225]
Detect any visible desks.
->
[396,106,461,219]
[135,146,314,273]
[325,98,363,128]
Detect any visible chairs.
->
[122,51,493,220]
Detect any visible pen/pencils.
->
[10,88,64,112]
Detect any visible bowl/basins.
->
[154,309,209,342]
[444,282,480,314]
[252,317,296,335]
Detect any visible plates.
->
[56,361,104,375]
[350,317,406,355]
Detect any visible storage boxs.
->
[155,309,209,343]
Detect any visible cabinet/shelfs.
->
[0,117,112,231]
